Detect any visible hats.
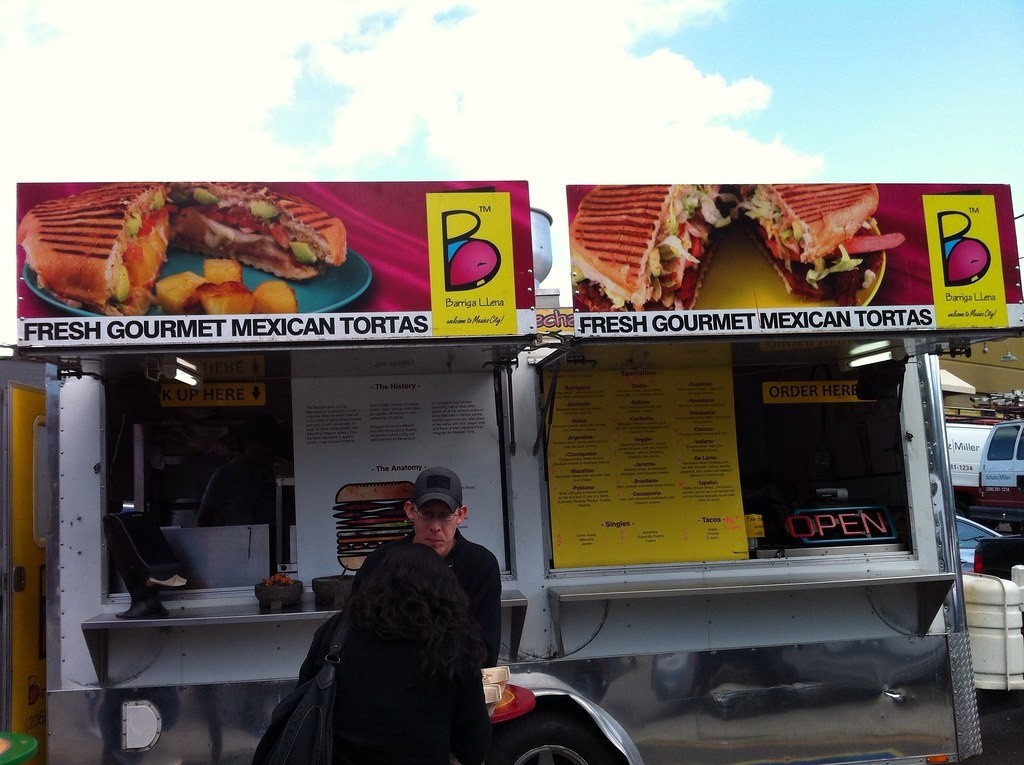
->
[411,466,463,514]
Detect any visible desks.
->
[546,570,959,659]
[80,588,530,691]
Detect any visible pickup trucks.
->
[937,366,1002,531]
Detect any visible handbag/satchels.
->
[252,607,355,765]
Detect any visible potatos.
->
[153,259,299,314]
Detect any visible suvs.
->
[968,419,1024,535]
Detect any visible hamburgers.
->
[16,183,179,318]
[162,182,347,279]
[728,184,906,306]
[570,184,734,312]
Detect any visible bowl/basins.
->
[254,579,303,607]
[312,575,356,603]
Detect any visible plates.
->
[696,218,885,307]
[21,247,371,315]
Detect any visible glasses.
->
[413,506,461,521]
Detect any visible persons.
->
[251,465,501,765]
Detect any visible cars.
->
[953,513,1024,580]
